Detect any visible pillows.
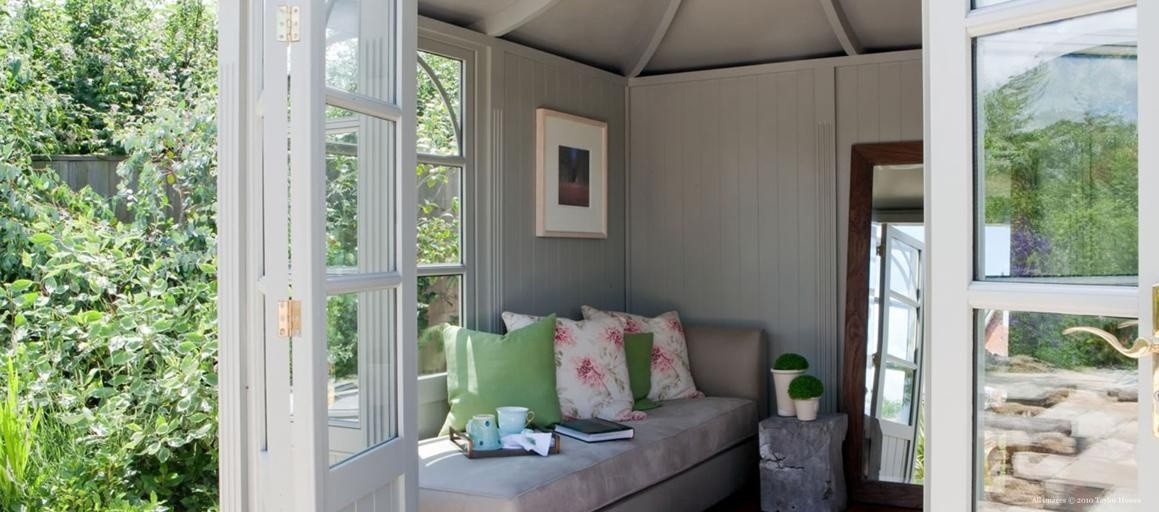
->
[624,332,663,410]
[500,312,647,421]
[579,305,706,405]
[437,312,564,438]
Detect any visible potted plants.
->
[787,374,824,422]
[770,352,809,416]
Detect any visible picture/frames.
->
[535,107,610,241]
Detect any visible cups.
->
[467,406,535,451]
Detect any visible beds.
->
[419,324,767,512]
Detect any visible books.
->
[553,415,634,442]
